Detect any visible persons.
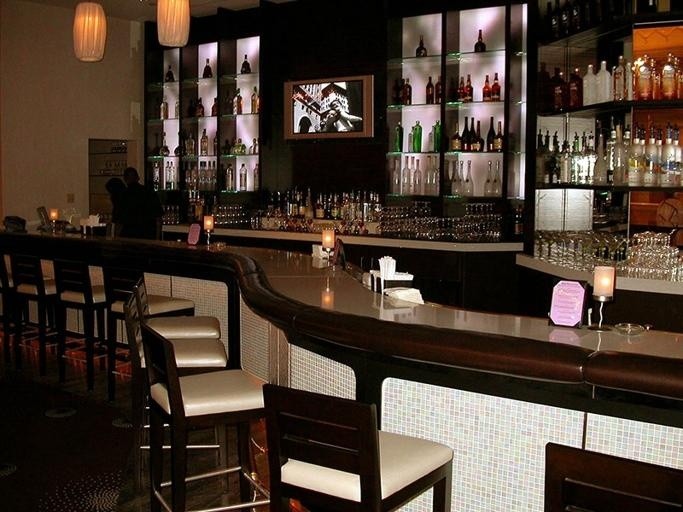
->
[112,167,164,242]
[104,178,126,236]
[330,97,361,131]
[309,110,338,133]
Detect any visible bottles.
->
[240,54,251,74]
[202,59,212,79]
[157,91,261,193]
[416,34,428,57]
[474,29,485,52]
[391,117,502,198]
[535,119,681,186]
[395,74,441,105]
[548,52,676,110]
[513,206,524,234]
[543,1,592,42]
[456,71,500,101]
[264,184,381,222]
[164,65,174,82]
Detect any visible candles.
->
[48,208,58,220]
[320,291,335,309]
[593,266,615,297]
[203,216,214,229]
[321,230,334,248]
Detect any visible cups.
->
[620,230,682,280]
[249,215,361,236]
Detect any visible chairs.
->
[0,250,196,398]
[123,292,230,494]
[263,383,454,512]
[131,278,222,465]
[545,440,682,511]
[140,321,265,512]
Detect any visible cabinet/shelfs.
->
[385,0,527,202]
[529,0,682,294]
[161,225,524,316]
[143,7,259,193]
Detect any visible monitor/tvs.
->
[284,75,374,140]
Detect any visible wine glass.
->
[104,159,127,174]
[161,203,247,224]
[381,200,501,240]
[536,225,624,270]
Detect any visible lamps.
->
[156,0,191,47]
[73,3,106,61]
